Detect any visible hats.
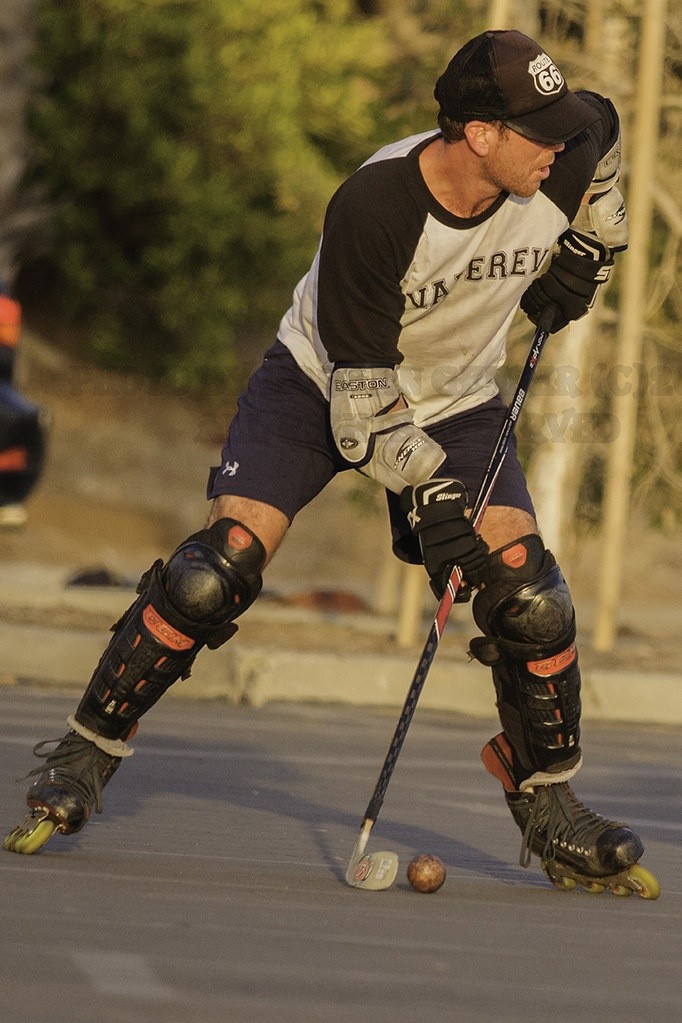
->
[435,27,598,148]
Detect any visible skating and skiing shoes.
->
[482,733,661,900]
[1,724,135,856]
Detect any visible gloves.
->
[398,476,490,601]
[518,225,623,340]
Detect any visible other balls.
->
[407,854,448,894]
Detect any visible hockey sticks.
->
[343,323,550,891]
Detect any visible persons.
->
[0,24,661,901]
[0,294,44,524]
